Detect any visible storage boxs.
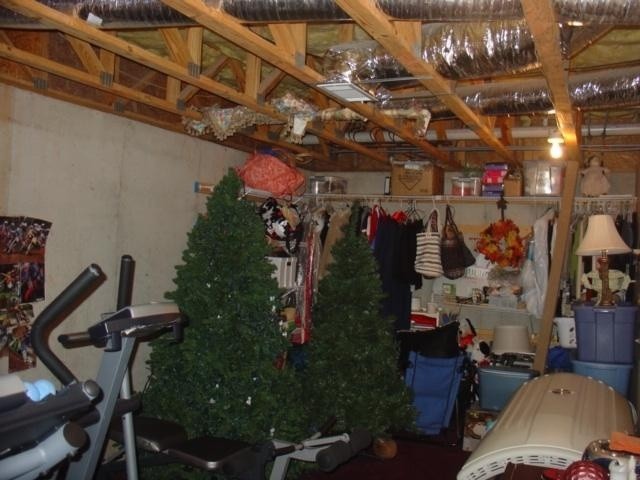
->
[391,163,445,196]
[569,300,638,398]
[475,365,540,410]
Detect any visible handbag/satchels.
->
[414,205,476,280]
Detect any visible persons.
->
[579,152,612,198]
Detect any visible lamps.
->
[575,215,633,306]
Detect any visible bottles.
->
[451,176,481,196]
